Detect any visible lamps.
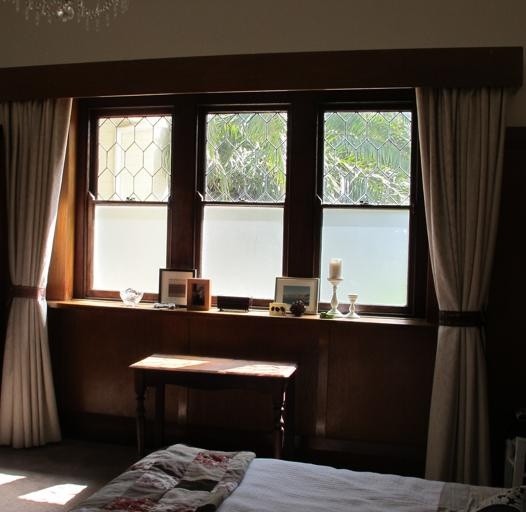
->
[0,0,130,32]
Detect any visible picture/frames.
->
[274,276,321,315]
[159,269,198,308]
[187,279,210,311]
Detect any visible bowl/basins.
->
[119,288,145,306]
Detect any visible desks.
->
[128,352,299,460]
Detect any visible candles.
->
[329,258,342,279]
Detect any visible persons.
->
[192,287,203,305]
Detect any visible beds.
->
[67,443,526,512]
